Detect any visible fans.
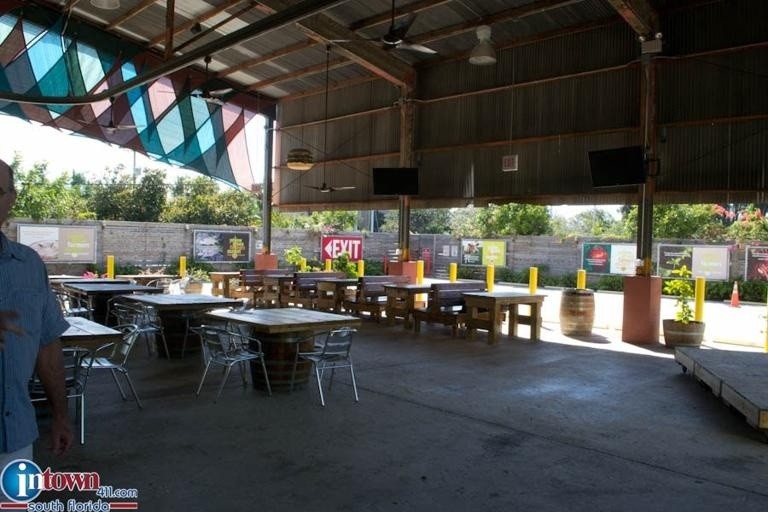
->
[327,0,440,58]
[75,93,147,138]
[156,51,236,106]
[303,45,357,194]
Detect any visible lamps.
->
[468,23,498,66]
[89,0,121,11]
[284,99,315,172]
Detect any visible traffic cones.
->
[729,281,741,307]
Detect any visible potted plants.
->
[662,246,706,351]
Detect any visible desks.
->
[27,269,551,450]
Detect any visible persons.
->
[0,159,72,501]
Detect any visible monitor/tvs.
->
[587,145,647,187]
[372,167,419,195]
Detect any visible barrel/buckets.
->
[559,288,594,337]
